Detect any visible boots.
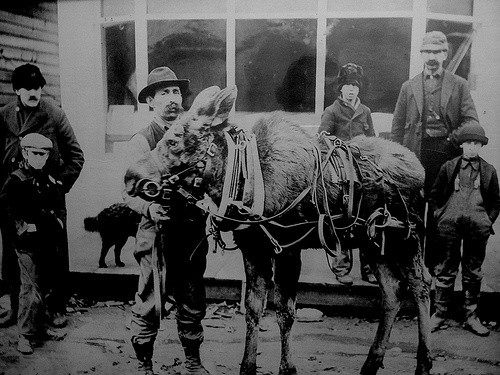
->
[462,281,490,336]
[430,285,455,333]
[131,336,157,375]
[179,334,210,375]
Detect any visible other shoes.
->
[0,310,17,327]
[363,273,378,284]
[16,337,33,353]
[49,312,66,328]
[335,274,353,284]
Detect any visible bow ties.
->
[426,74,440,79]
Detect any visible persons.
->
[0,63,85,354]
[388,30,500,336]
[120,67,239,375]
[317,63,378,285]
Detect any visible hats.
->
[420,30,449,52]
[452,122,488,147]
[20,132,53,149]
[336,63,363,90]
[12,64,47,89]
[138,67,190,104]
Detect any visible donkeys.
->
[122,83,435,375]
